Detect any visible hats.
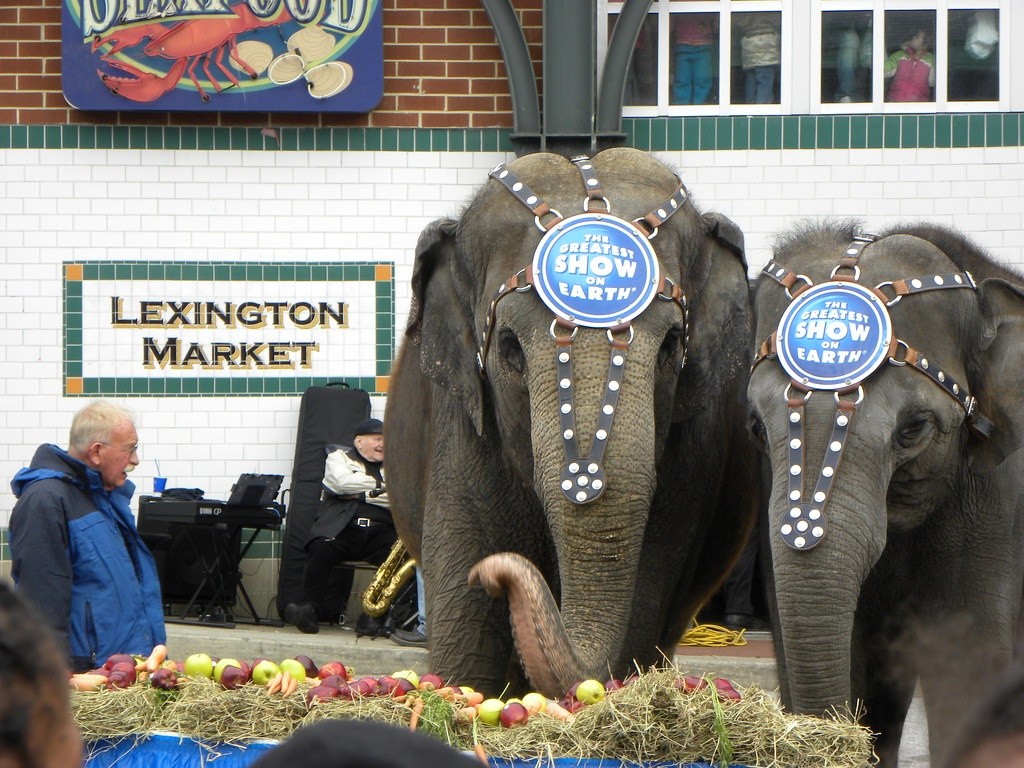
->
[354,419,385,435]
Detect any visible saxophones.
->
[362,534,416,618]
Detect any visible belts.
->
[349,518,389,528]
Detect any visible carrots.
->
[475,744,489,768]
[546,701,572,719]
[69,645,167,691]
[392,682,483,731]
[266,672,322,698]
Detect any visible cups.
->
[153,477,167,497]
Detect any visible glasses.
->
[102,445,138,457]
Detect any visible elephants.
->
[745,216,1024,768]
[383,146,762,703]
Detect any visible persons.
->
[608,12,780,106]
[0,578,86,768]
[7,399,170,675]
[830,10,936,102]
[283,419,411,637]
[391,563,429,647]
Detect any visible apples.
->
[177,653,319,690]
[682,676,741,703]
[557,676,639,713]
[420,674,547,728]
[89,654,176,691]
[306,661,419,704]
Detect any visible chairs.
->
[318,444,380,571]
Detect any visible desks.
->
[146,516,283,629]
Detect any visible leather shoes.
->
[390,628,429,647]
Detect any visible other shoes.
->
[726,614,752,629]
[284,602,319,633]
[355,613,385,634]
